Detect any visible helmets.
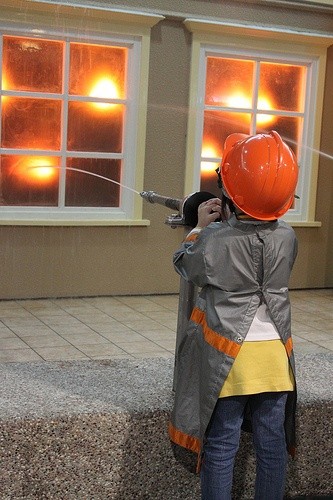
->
[220,130,300,220]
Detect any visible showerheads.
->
[137,190,183,213]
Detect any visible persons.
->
[172,128,299,500]
